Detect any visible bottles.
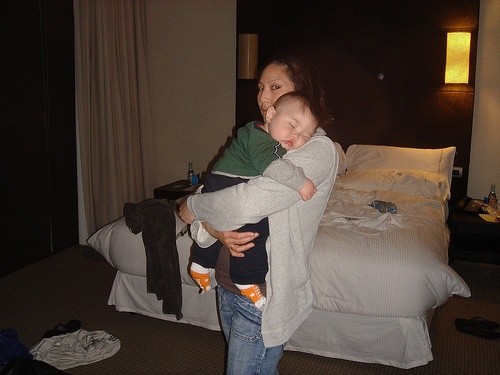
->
[486,183,497,206]
[188,162,194,185]
[366,201,398,214]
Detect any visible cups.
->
[192,173,199,184]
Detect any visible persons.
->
[189,91,323,308]
[179,56,340,375]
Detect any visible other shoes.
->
[455,316,500,339]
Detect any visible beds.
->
[87,167,471,368]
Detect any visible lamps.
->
[238,33,258,79]
[445,32,471,83]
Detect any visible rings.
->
[230,244,234,248]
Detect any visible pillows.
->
[345,144,457,200]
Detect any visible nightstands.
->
[153,179,202,200]
[454,199,500,266]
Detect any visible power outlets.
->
[453,167,463,178]
